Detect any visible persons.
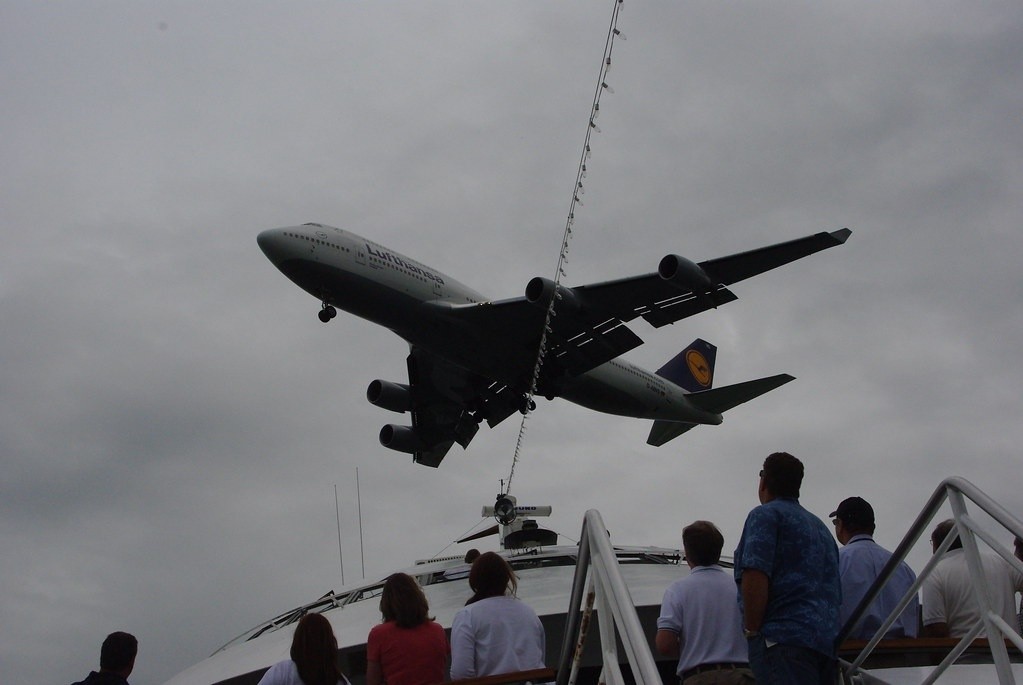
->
[734,452,844,684]
[828,496,920,641]
[364,571,450,685]
[923,519,1021,664]
[450,549,556,685]
[71,631,139,685]
[258,613,352,685]
[656,520,757,685]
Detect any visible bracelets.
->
[743,627,758,637]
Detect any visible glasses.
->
[929,539,934,545]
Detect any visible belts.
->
[682,662,751,681]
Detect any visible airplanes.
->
[254,222,855,474]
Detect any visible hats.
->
[829,497,873,519]
